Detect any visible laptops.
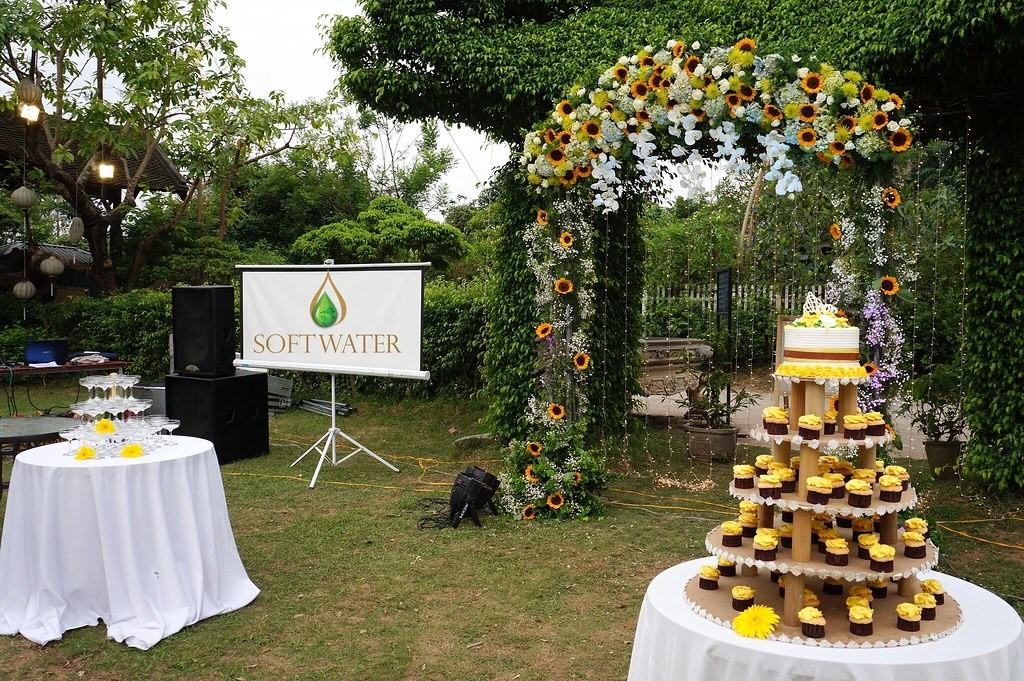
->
[17,339,69,366]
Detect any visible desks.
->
[0,433,261,652]
[0,360,134,420]
[0,416,87,501]
[623,555,1024,681]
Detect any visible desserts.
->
[732,455,910,508]
[721,500,928,572]
[698,557,944,639]
[761,406,886,441]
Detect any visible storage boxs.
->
[132,385,168,436]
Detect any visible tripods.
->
[299,399,357,418]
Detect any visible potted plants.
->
[669,345,764,463]
[896,361,968,480]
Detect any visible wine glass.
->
[60,376,180,457]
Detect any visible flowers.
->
[74,444,97,461]
[731,603,781,641]
[94,417,115,435]
[484,36,915,525]
[120,443,144,459]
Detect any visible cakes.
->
[773,291,868,379]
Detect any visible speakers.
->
[172,285,236,378]
[164,371,270,465]
